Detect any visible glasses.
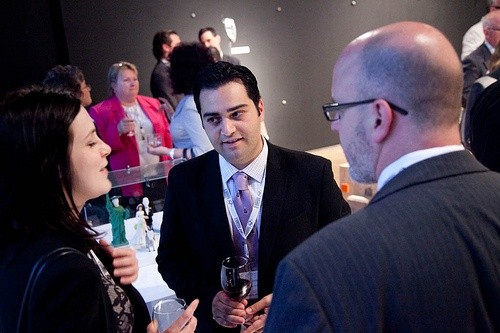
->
[322,99,408,122]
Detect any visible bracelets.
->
[169,148,176,159]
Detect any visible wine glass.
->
[123,109,136,136]
[220,255,254,323]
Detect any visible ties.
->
[232,171,259,271]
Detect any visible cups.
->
[153,298,188,333]
[146,134,162,148]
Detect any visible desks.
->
[91,210,178,317]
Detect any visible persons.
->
[461,0,500,61]
[0,87,199,333]
[149,30,185,111]
[88,62,173,201]
[42,64,92,106]
[263,20,500,333]
[155,61,352,333]
[146,41,217,161]
[134,204,148,244]
[460,10,500,109]
[199,27,240,65]
[104,193,131,246]
[141,197,154,231]
[464,41,500,174]
